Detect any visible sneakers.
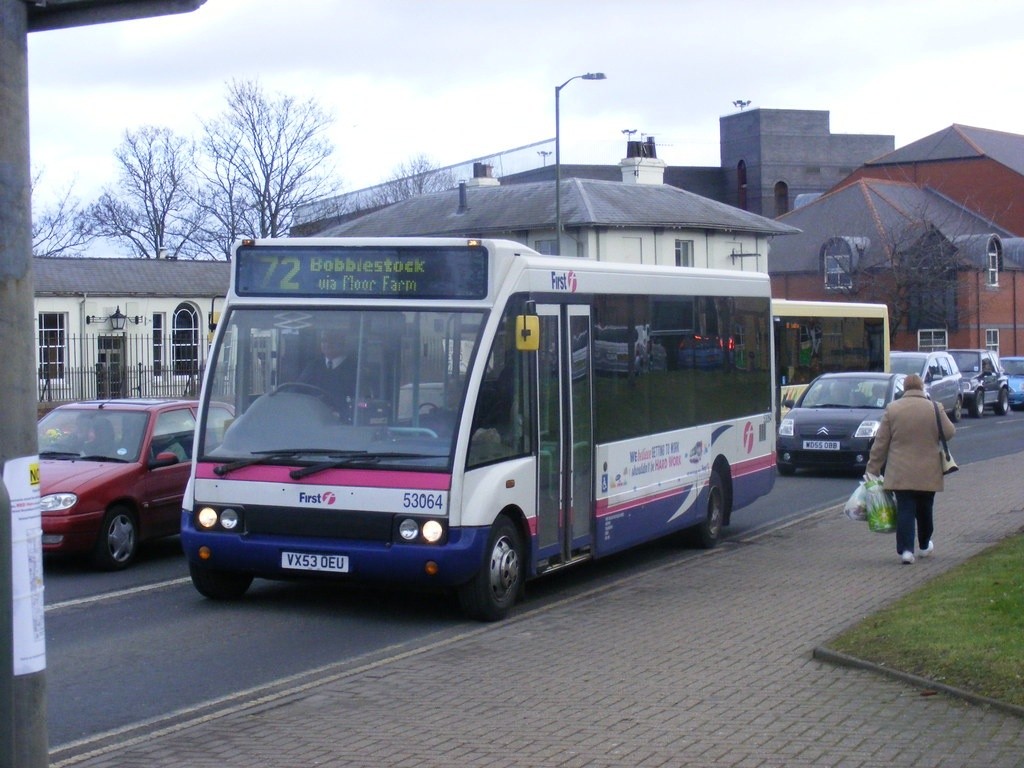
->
[919,540,934,557]
[902,550,915,564]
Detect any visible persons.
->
[300,329,368,426]
[75,413,96,443]
[137,420,187,464]
[864,374,956,563]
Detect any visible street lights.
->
[555,74,608,258]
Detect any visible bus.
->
[769,299,891,423]
[178,234,773,619]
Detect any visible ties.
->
[327,359,333,368]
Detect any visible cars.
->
[1000,357,1024,407]
[776,371,929,476]
[570,330,589,380]
[681,345,725,366]
[37,398,243,571]
[593,325,648,376]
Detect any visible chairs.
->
[96,416,115,442]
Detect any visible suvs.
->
[946,348,1010,417]
[890,351,965,422]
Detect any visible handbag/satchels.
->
[940,448,959,475]
[844,472,897,532]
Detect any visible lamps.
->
[110,305,126,330]
